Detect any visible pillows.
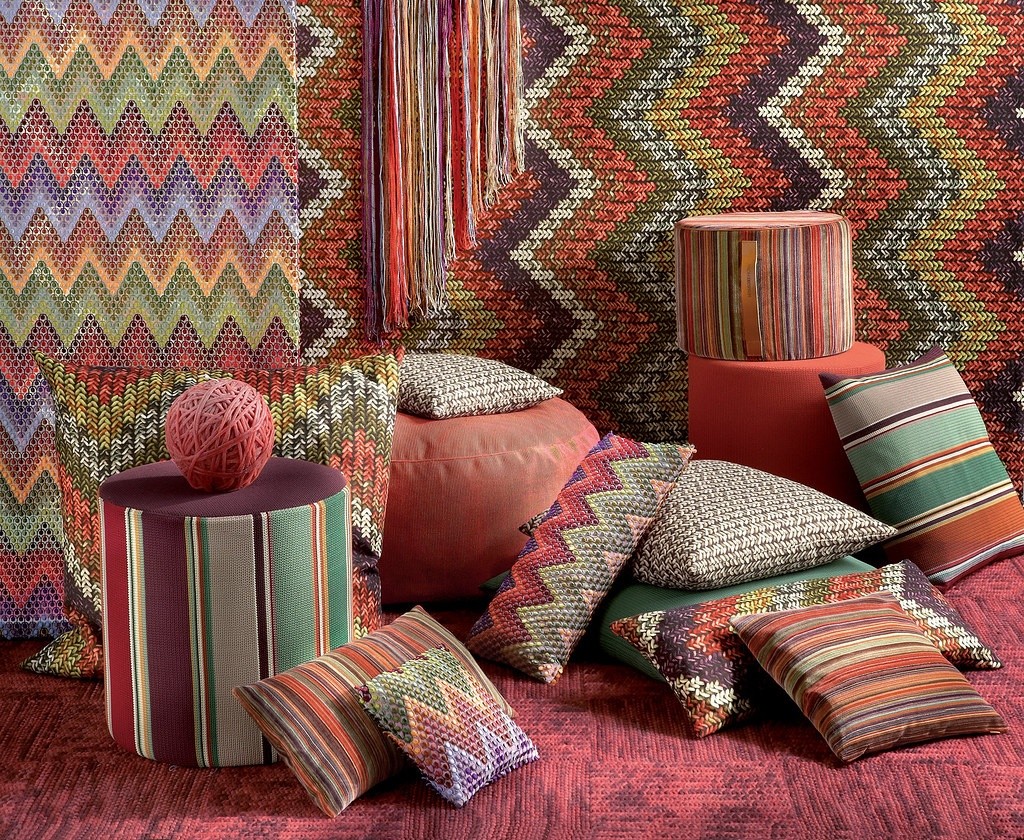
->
[594,553,874,680]
[395,353,565,420]
[620,457,898,590]
[730,594,1011,760]
[612,559,1006,738]
[357,644,539,811]
[467,432,695,683]
[21,355,400,697]
[234,605,523,815]
[817,357,1022,583]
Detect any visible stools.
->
[677,213,856,359]
[100,462,355,764]
[378,404,603,607]
[688,345,885,504]
